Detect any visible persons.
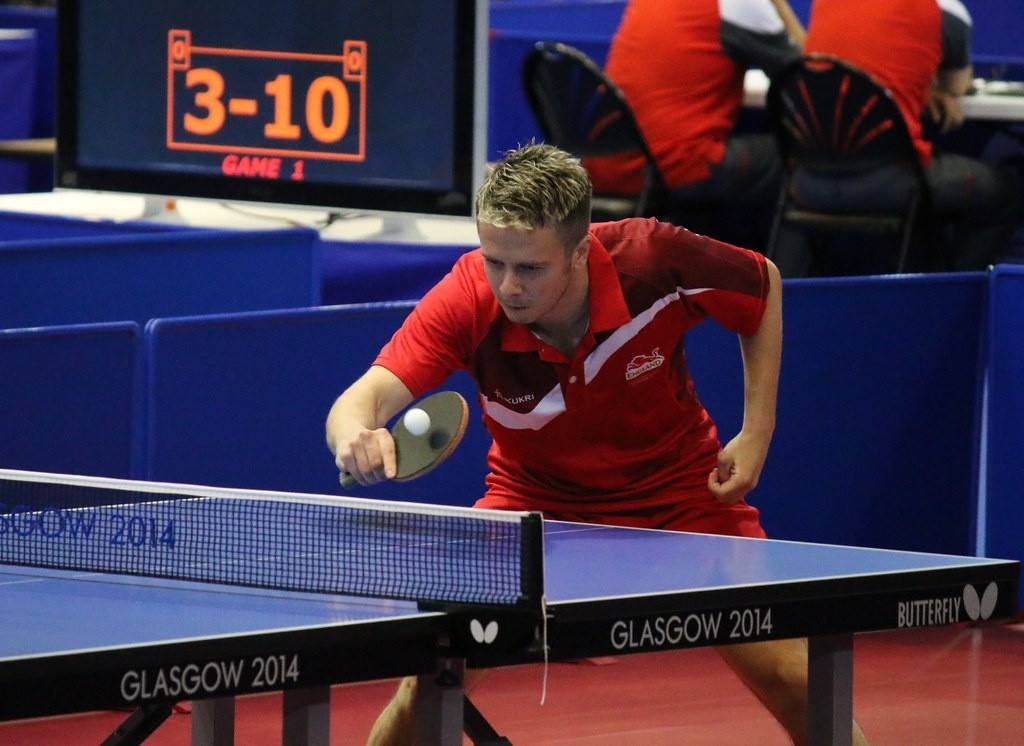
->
[326,143,871,746]
[580,0,999,210]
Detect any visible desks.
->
[0,469,1022,746]
[746,67,1024,122]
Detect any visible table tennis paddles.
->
[338,389,470,490]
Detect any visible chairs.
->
[753,54,941,277]
[521,39,694,222]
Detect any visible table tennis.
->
[403,407,432,437]
